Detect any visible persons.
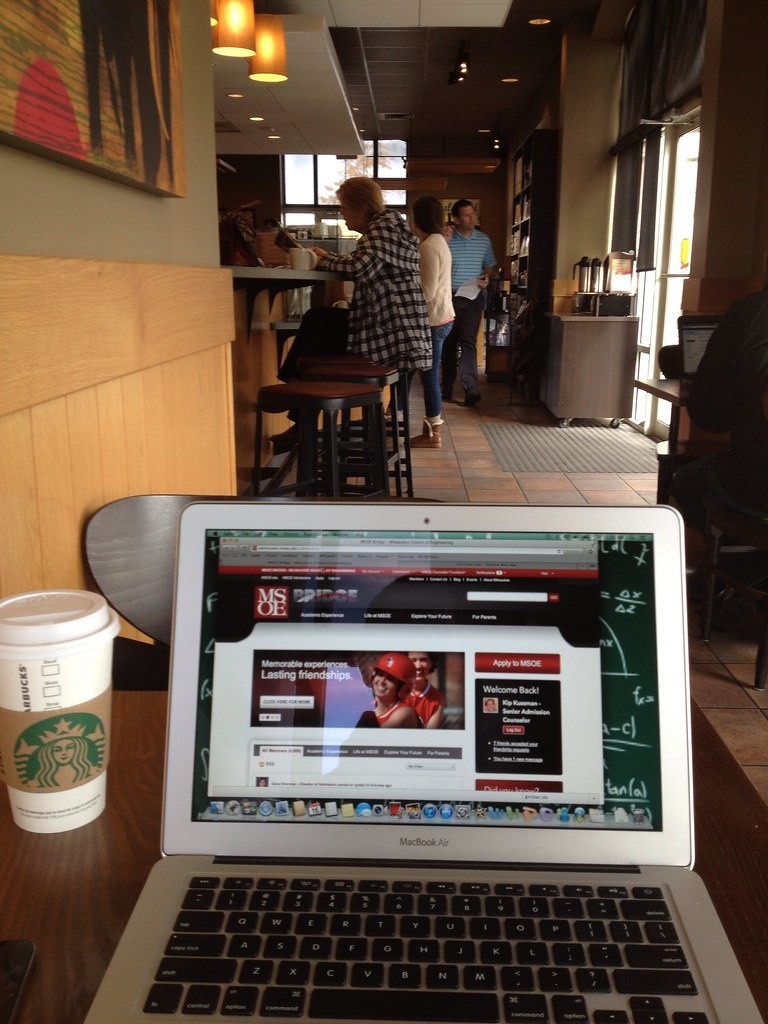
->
[364,652,446,729]
[439,199,497,406]
[265,177,434,443]
[671,288,768,629]
[483,698,498,713]
[384,197,457,447]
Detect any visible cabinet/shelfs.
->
[506,127,561,383]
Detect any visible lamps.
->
[210,0,256,58]
[248,13,288,83]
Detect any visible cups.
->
[311,222,328,238]
[289,248,317,271]
[0,589,123,835]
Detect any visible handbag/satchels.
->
[219,199,262,268]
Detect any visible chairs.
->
[698,480,768,692]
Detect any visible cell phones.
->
[0,939,37,1024]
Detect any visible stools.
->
[253,355,413,497]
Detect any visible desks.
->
[1,690,768,1024]
[222,265,354,347]
[539,313,639,418]
[632,371,735,506]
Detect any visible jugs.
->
[591,257,604,293]
[573,256,591,292]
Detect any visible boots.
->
[404,418,444,447]
[383,414,393,432]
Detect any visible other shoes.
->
[268,426,318,442]
[440,380,454,402]
[464,389,481,405]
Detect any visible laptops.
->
[84,500,766,1024]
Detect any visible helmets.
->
[372,652,417,685]
